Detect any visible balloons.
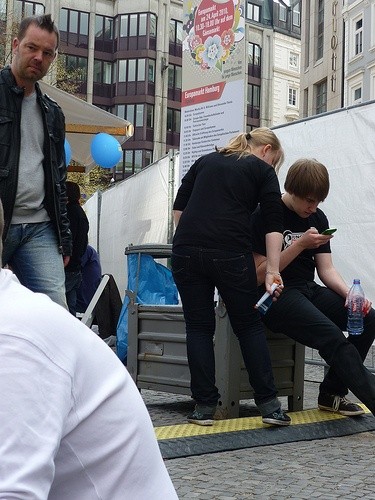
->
[63,137,71,168]
[91,132,123,169]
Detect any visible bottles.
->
[348,279,364,334]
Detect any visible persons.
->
[0,200,180,500]
[62,181,101,319]
[248,158,375,417]
[0,14,73,316]
[170,127,291,427]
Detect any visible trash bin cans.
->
[124,242,307,420]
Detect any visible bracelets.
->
[264,272,280,276]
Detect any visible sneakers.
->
[318,393,365,415]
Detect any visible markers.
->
[254,279,282,310]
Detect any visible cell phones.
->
[322,228,338,235]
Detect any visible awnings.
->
[36,80,133,174]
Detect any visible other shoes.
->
[186,411,214,426]
[262,408,291,425]
